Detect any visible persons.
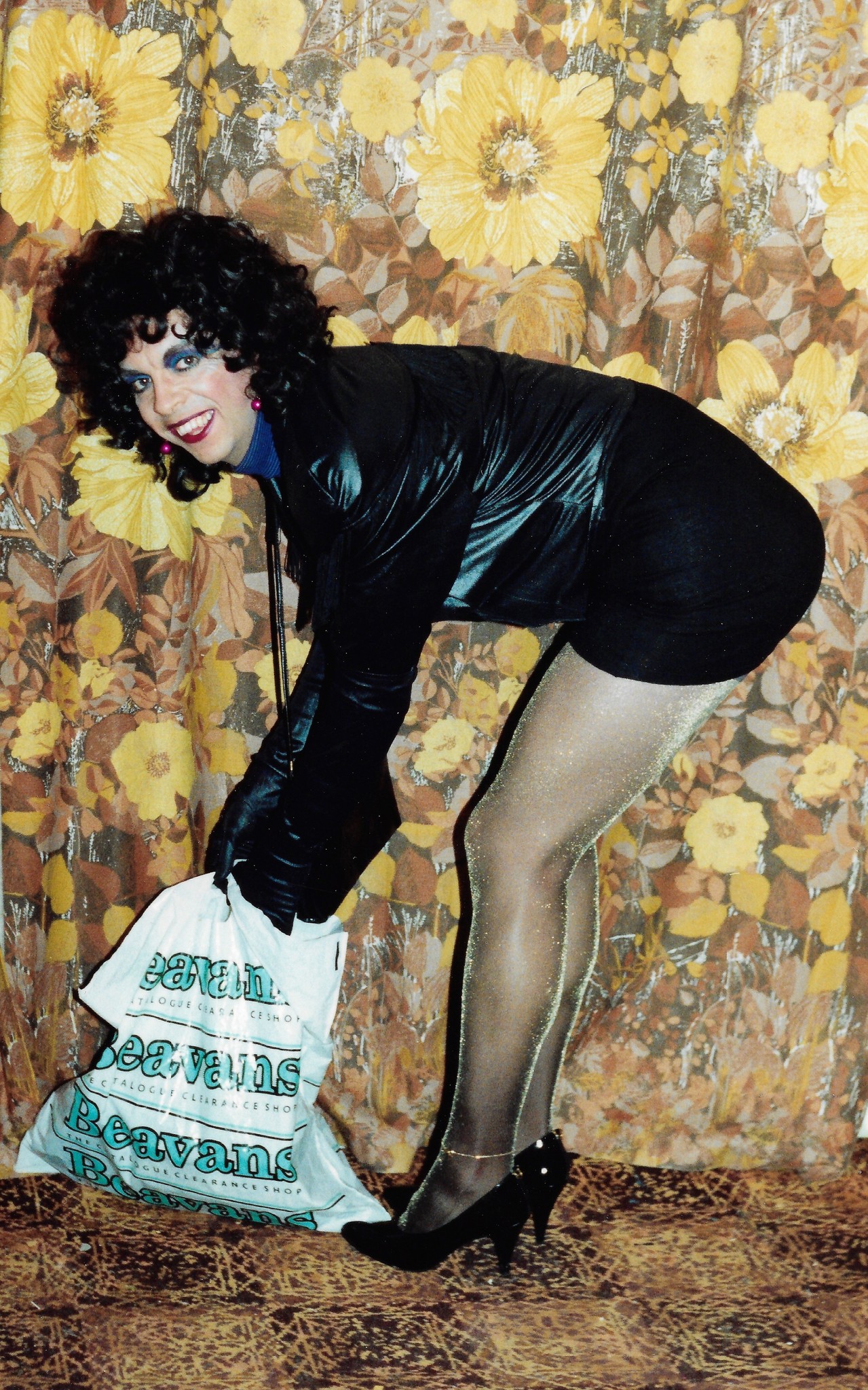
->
[47,205,826,1279]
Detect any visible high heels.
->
[384,1130,574,1248]
[340,1168,534,1275]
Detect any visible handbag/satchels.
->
[19,857,394,1235]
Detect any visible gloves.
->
[200,746,403,934]
